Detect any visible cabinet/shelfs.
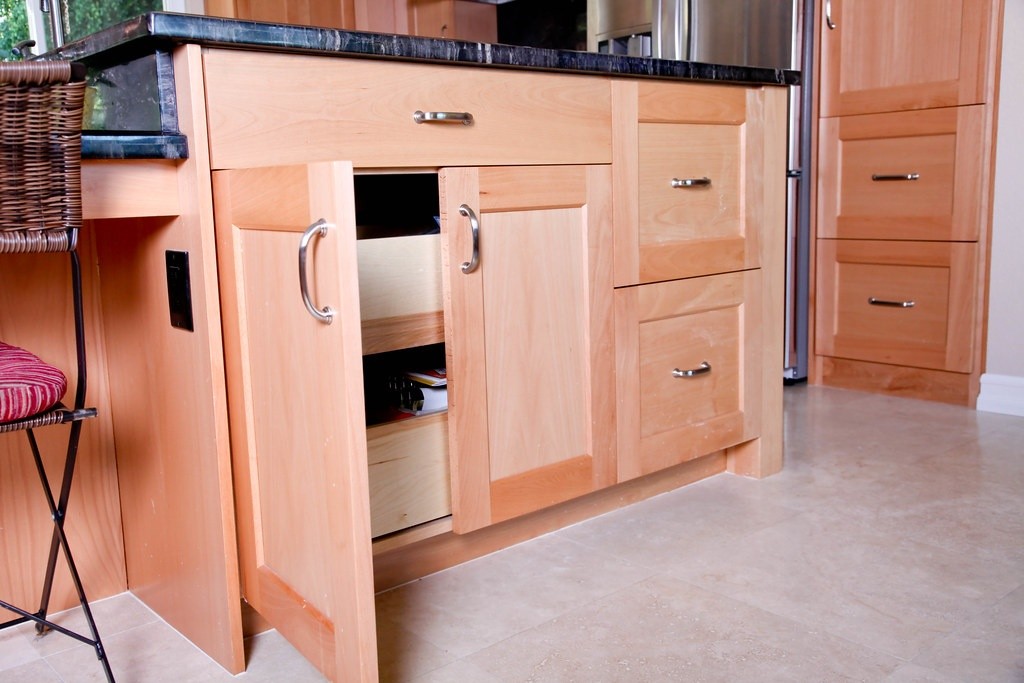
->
[0,40,789,683]
[808,0,1005,408]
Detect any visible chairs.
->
[0,63,118,683]
